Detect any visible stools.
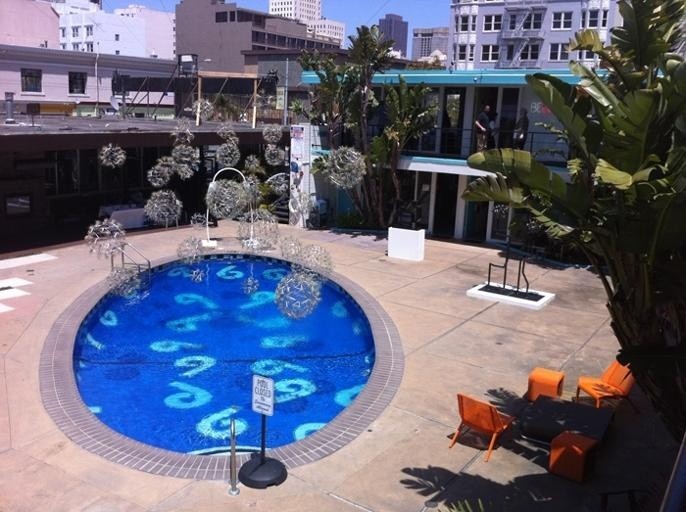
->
[547,431,601,486]
[527,366,565,401]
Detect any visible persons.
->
[474,103,490,151]
[488,111,499,148]
[513,107,529,150]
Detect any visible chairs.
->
[447,393,518,463]
[574,359,637,420]
